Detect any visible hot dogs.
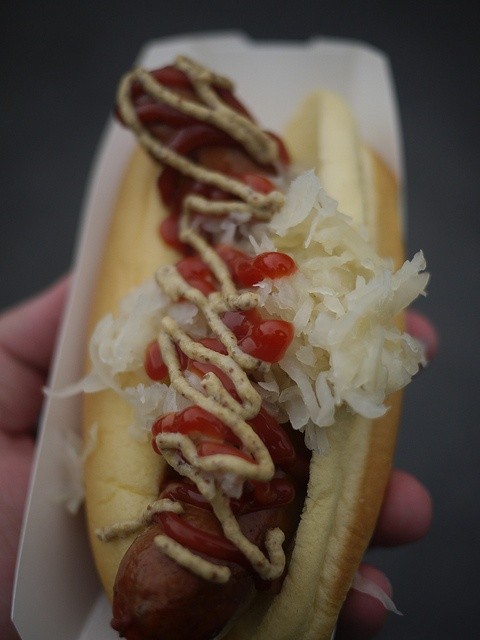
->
[75,54,431,638]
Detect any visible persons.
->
[0,274,439,640]
